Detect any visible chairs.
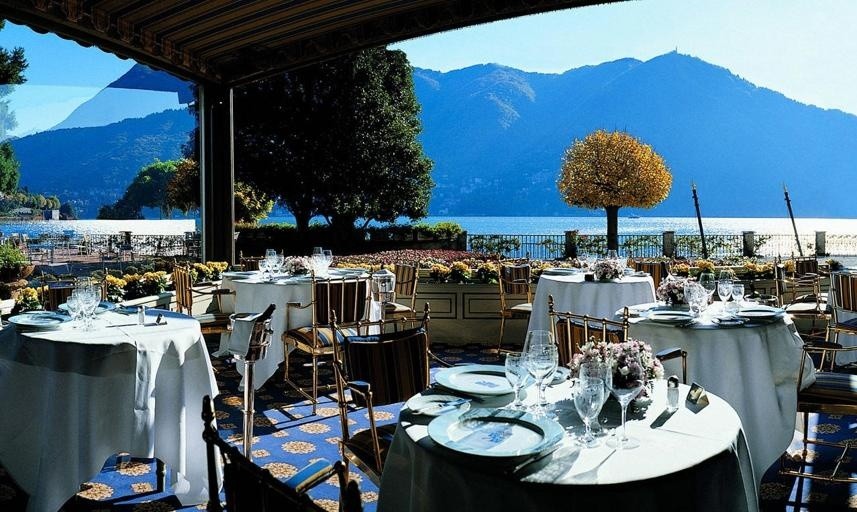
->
[1,245,856,511]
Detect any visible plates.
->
[408,364,565,458]
[544,263,633,276]
[8,301,115,326]
[649,307,776,326]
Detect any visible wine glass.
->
[505,330,642,450]
[67,275,102,335]
[258,246,333,281]
[683,270,745,320]
[578,249,627,277]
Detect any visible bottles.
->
[138,305,145,325]
[667,375,679,409]
